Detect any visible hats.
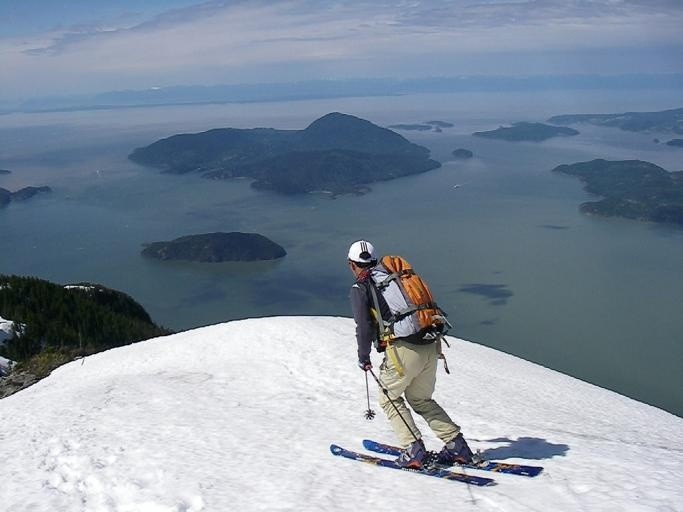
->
[348,239,376,264]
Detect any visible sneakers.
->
[434,435,474,464]
[393,438,428,470]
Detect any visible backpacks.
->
[352,252,448,346]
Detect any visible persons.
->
[346,239,474,469]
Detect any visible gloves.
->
[357,360,373,372]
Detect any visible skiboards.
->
[329,440,543,486]
